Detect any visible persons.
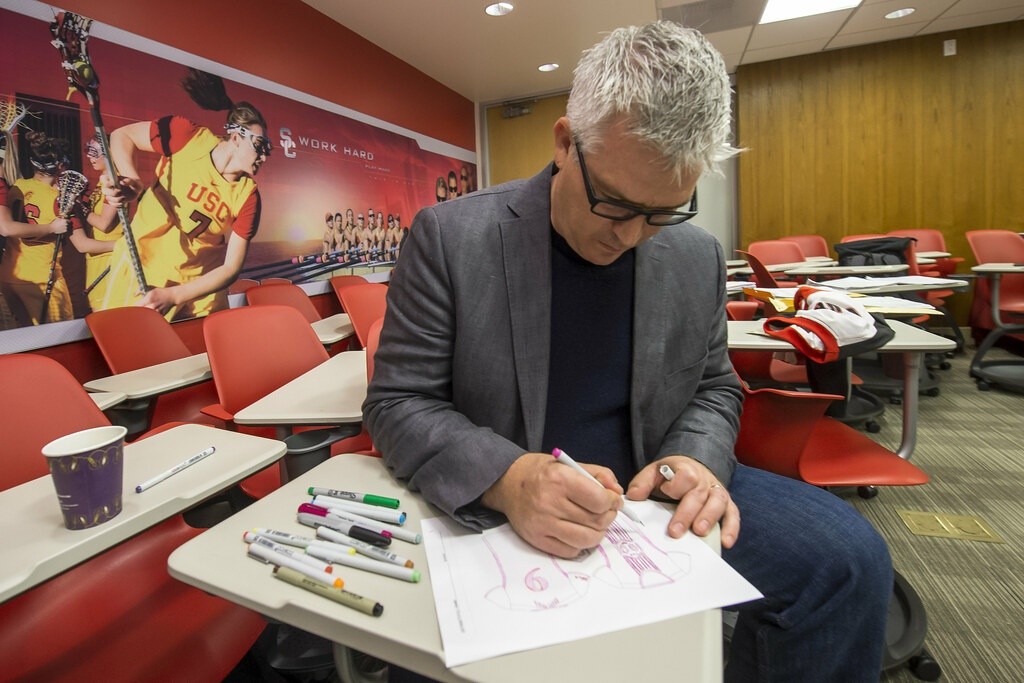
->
[361,19,895,683]
[350,212,367,260]
[434,177,447,203]
[0,130,127,328]
[362,208,377,262]
[447,170,461,200]
[98,68,274,325]
[384,214,404,259]
[321,212,335,263]
[458,164,472,197]
[343,208,359,262]
[334,213,345,264]
[372,211,386,261]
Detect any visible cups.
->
[40,425,128,531]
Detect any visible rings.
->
[710,484,722,488]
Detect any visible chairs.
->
[726,229,1024,499]
[292,250,400,262]
[0,275,389,683]
[228,278,292,294]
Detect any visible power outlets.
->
[943,39,956,56]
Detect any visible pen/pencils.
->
[297,512,392,546]
[247,543,344,590]
[308,487,400,508]
[327,508,421,544]
[315,527,414,568]
[135,447,217,493]
[310,494,406,524]
[242,532,333,574]
[298,503,391,538]
[252,528,357,555]
[273,565,384,617]
[305,546,420,583]
[551,448,645,527]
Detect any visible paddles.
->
[239,247,399,284]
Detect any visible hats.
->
[325,213,333,222]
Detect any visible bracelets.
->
[351,245,354,247]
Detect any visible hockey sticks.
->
[49,10,149,296]
[38,169,90,325]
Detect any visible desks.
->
[916,258,937,264]
[727,318,958,498]
[240,255,396,284]
[726,259,747,268]
[916,250,952,259]
[785,264,910,277]
[726,282,758,298]
[798,274,969,405]
[754,287,947,432]
[736,262,838,274]
[969,260,1024,394]
[804,256,833,261]
[0,296,937,683]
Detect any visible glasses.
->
[388,219,393,223]
[460,175,467,180]
[369,214,374,217]
[85,144,99,158]
[449,187,457,192]
[358,218,364,220]
[574,134,699,227]
[437,196,446,202]
[250,133,270,156]
